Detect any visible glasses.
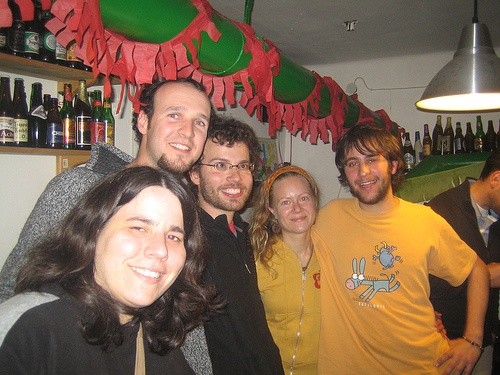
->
[193,162,254,175]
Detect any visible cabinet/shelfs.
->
[0,52,121,156]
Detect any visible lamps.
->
[415,0,500,113]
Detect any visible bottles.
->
[403,116,500,172]
[0,0,115,150]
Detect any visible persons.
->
[309,123,488,375]
[248,165,321,375]
[425,149,500,375]
[0,77,214,375]
[0,165,224,375]
[186,119,285,375]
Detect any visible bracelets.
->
[462,336,484,352]
